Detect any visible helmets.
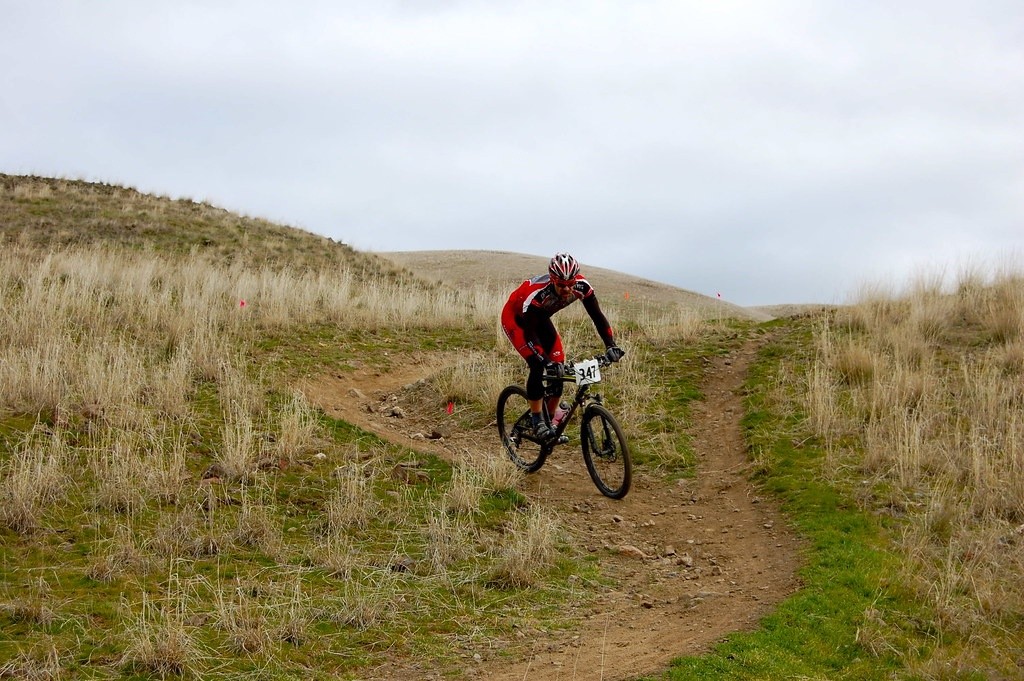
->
[548,254,581,281]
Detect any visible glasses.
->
[554,280,576,288]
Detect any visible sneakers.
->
[550,424,569,444]
[532,421,556,441]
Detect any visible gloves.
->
[605,343,622,362]
[544,360,565,378]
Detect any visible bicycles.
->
[495,350,632,500]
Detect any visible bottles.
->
[552,401,569,427]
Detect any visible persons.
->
[501,251,622,444]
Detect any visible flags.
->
[240,301,244,307]
[447,403,453,413]
[718,294,720,297]
